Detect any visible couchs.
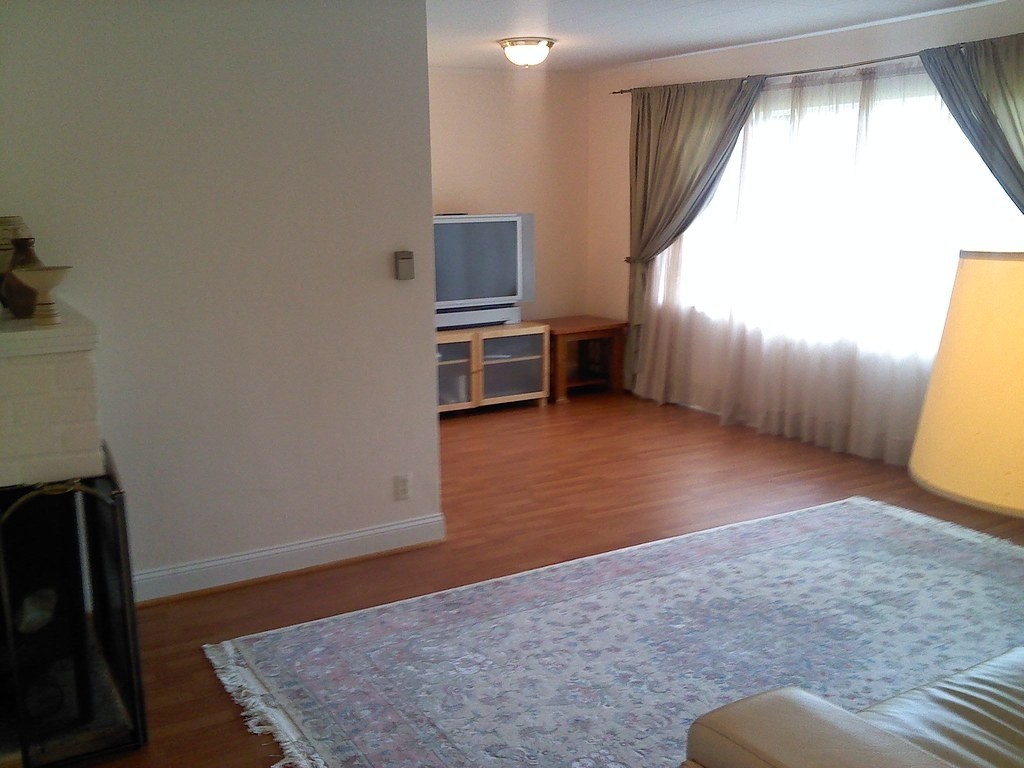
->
[676,641,1024,768]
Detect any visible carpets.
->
[201,494,1024,768]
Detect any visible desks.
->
[528,314,628,406]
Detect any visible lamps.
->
[907,244,1024,520]
[497,37,556,67]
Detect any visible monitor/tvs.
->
[433,214,522,310]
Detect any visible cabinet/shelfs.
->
[436,321,550,414]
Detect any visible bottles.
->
[0,216,34,307]
[2,236,45,318]
